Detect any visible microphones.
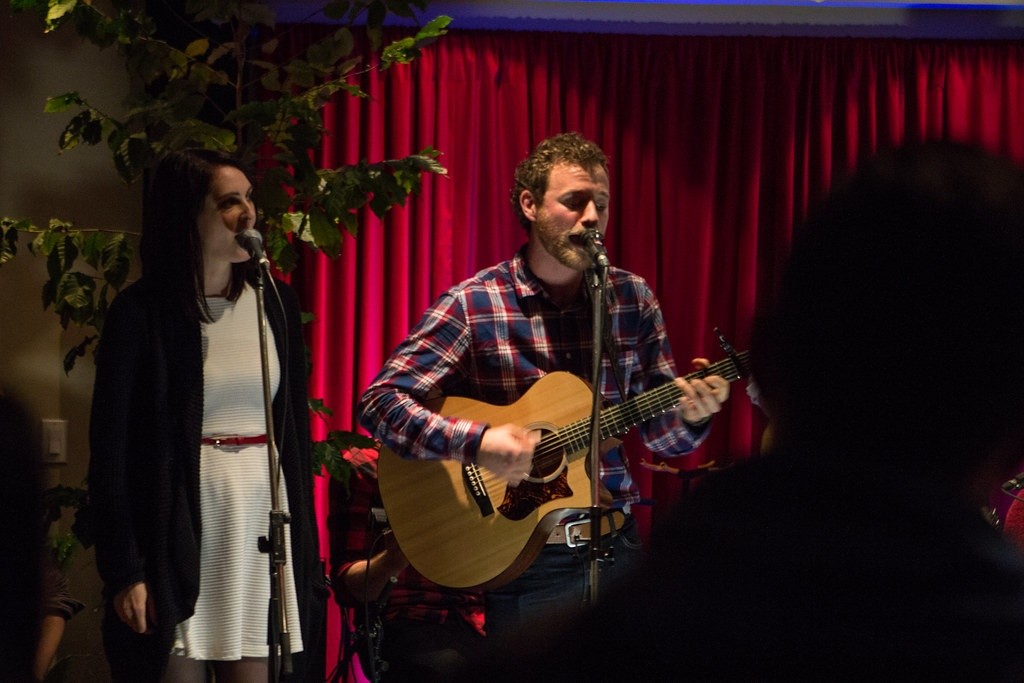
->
[236,228,269,273]
[580,227,611,268]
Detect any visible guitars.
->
[377,322,751,593]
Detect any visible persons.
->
[355,131,730,683]
[445,137,1024,683]
[88,145,317,683]
[0,380,88,683]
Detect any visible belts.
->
[545,509,630,548]
[201,435,270,447]
